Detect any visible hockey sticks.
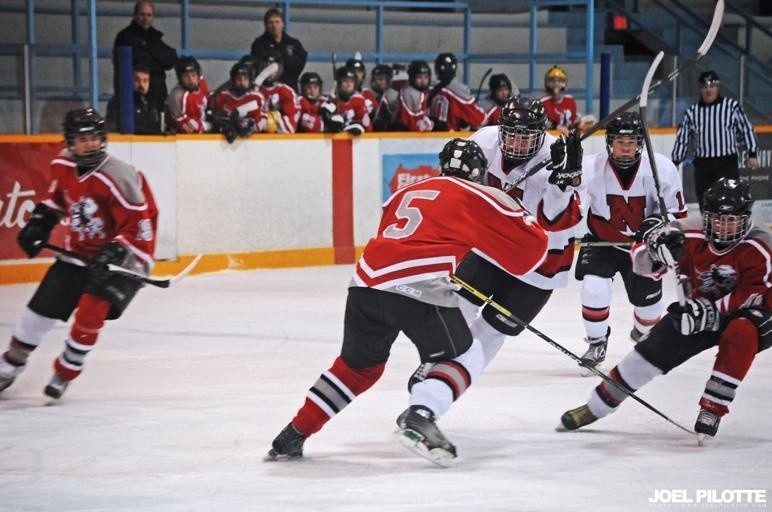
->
[502,0,726,194]
[639,50,689,306]
[32,239,203,288]
[451,274,697,437]
[251,63,278,94]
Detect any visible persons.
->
[408,96,593,393]
[671,71,757,215]
[560,176,772,436]
[105,0,577,143]
[0,108,158,401]
[579,111,688,366]
[272,138,549,459]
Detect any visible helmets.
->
[606,112,644,169]
[408,53,456,90]
[438,139,487,181]
[300,73,322,102]
[335,59,393,95]
[698,70,719,86]
[62,108,106,168]
[545,65,568,92]
[498,95,547,161]
[703,177,753,243]
[489,74,511,92]
[230,54,284,88]
[176,56,199,80]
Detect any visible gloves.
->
[635,214,685,266]
[207,108,255,143]
[89,241,126,282]
[17,203,59,257]
[549,128,582,186]
[667,296,722,336]
[318,102,365,135]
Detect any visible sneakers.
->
[695,407,721,436]
[581,326,610,365]
[631,325,647,342]
[396,405,457,460]
[563,404,597,429]
[272,422,305,456]
[46,375,69,398]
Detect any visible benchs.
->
[618,0,771,126]
